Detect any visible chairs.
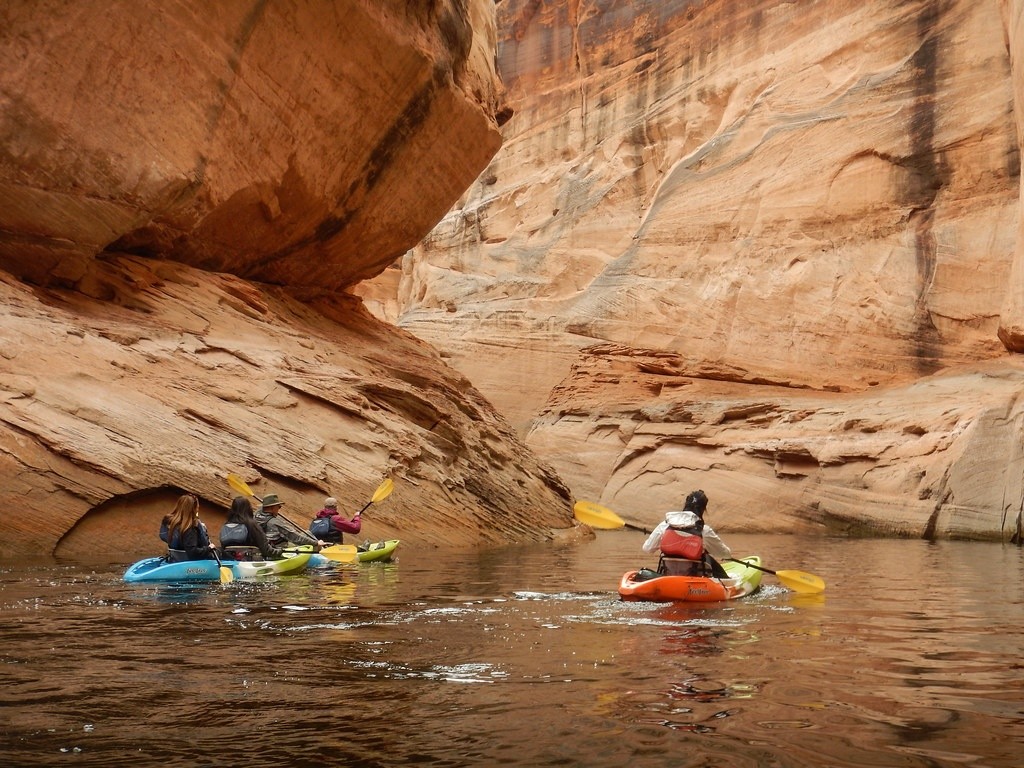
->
[225,545,263,560]
[661,556,713,577]
[169,549,188,562]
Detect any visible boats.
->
[614,602,764,627]
[617,554,762,602]
[313,538,401,562]
[135,562,405,603]
[122,545,313,582]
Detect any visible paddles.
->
[227,471,329,549]
[352,478,395,523]
[208,538,234,584]
[574,497,826,596]
[238,543,359,562]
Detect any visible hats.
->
[324,498,337,508]
[260,494,286,507]
[685,490,709,508]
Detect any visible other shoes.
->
[375,539,385,550]
[362,538,371,551]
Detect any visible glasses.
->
[274,505,282,509]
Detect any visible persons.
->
[253,495,326,548]
[221,496,283,561]
[316,498,385,552]
[167,493,235,561]
[643,490,732,579]
